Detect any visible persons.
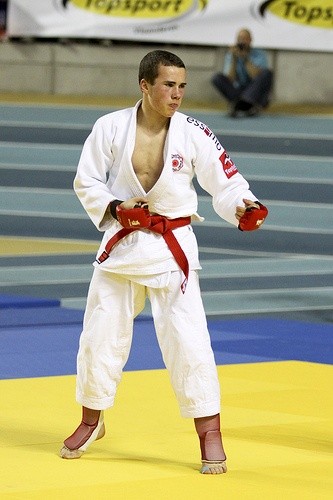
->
[211,30,274,118]
[59,50,269,474]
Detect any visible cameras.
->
[237,43,250,51]
